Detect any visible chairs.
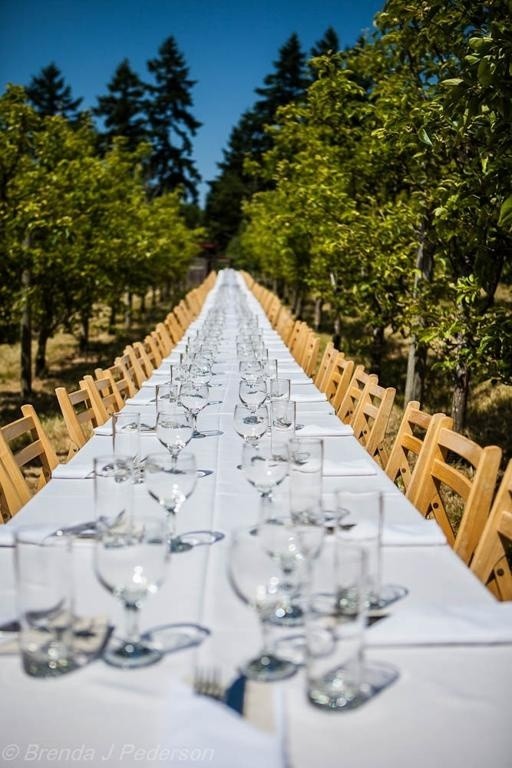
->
[1,272,512,604]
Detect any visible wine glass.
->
[228,335,390,712]
[13,335,228,677]
